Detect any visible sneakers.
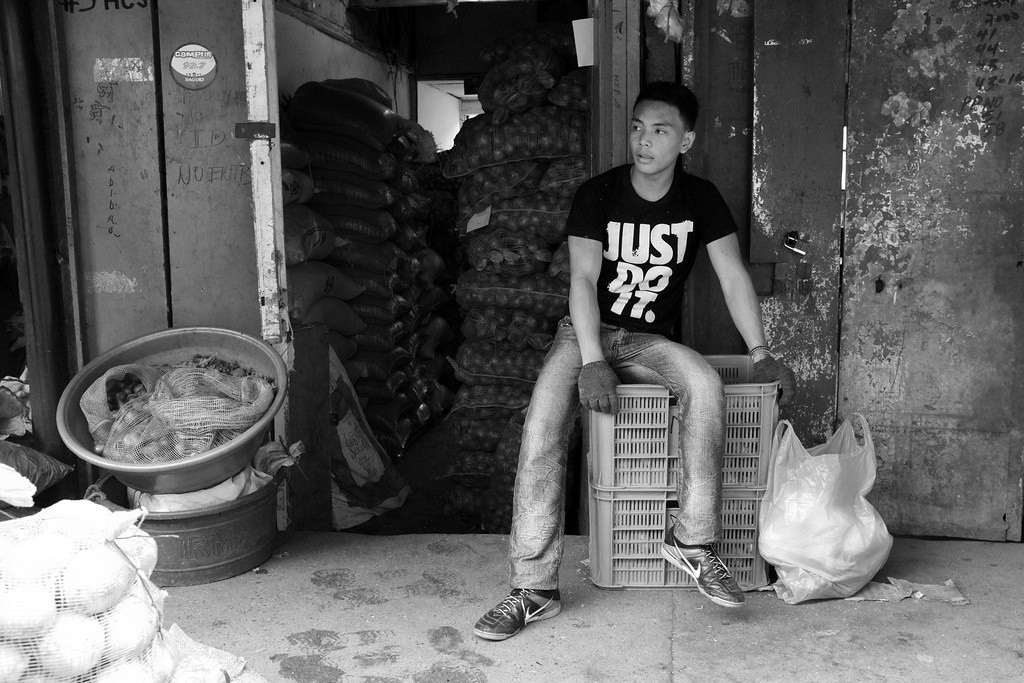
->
[472,586,561,640]
[661,522,747,609]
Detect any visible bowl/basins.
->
[56,327,287,494]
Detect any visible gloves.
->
[577,361,621,414]
[752,356,795,407]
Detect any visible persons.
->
[474,80,793,642]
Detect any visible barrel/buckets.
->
[91,466,289,587]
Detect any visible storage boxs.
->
[584,356,780,591]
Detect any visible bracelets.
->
[749,346,771,360]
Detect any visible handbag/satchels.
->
[757,413,892,604]
